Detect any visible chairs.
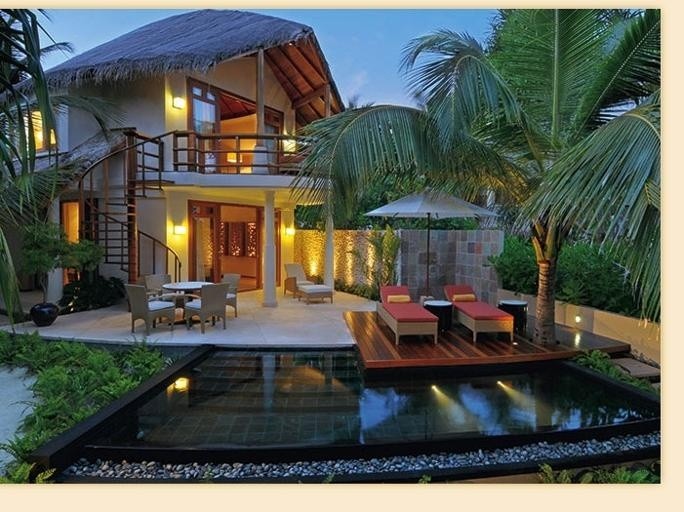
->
[376,285,440,345]
[444,283,515,343]
[282,263,313,301]
[144,274,177,322]
[184,282,228,333]
[123,283,176,335]
[220,272,241,320]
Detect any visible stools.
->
[298,285,333,305]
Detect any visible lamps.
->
[172,96,184,110]
[286,226,294,234]
[173,226,185,233]
[227,153,243,164]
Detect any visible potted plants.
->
[14,222,105,327]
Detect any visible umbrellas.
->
[362,182,501,297]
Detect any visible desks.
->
[498,297,529,338]
[161,281,213,329]
[423,298,453,334]
